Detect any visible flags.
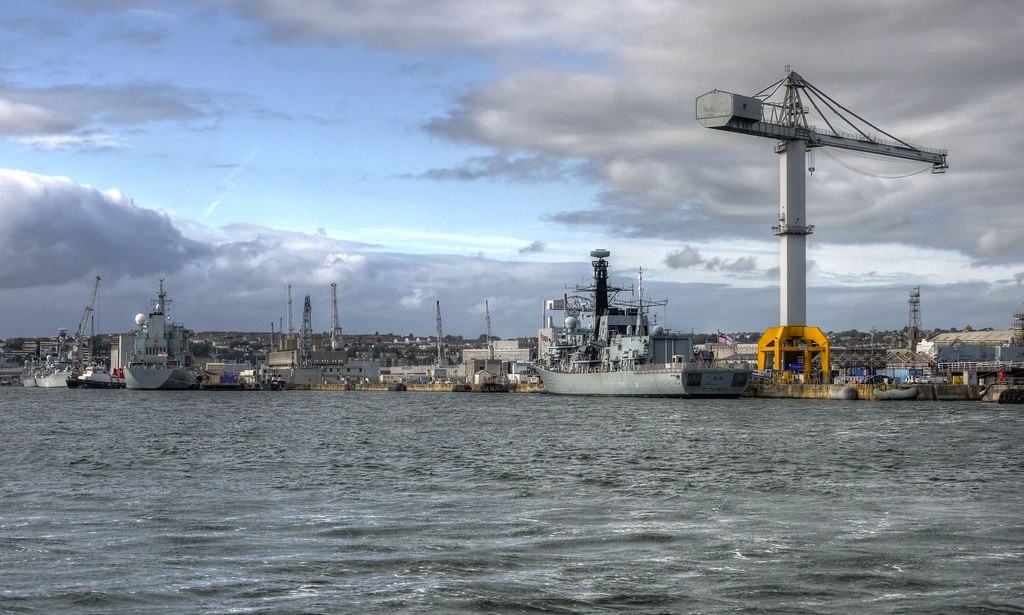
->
[541,334,548,342]
[719,332,735,346]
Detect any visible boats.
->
[24,246,1021,407]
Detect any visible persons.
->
[998,371,1004,384]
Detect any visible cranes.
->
[693,71,952,399]
